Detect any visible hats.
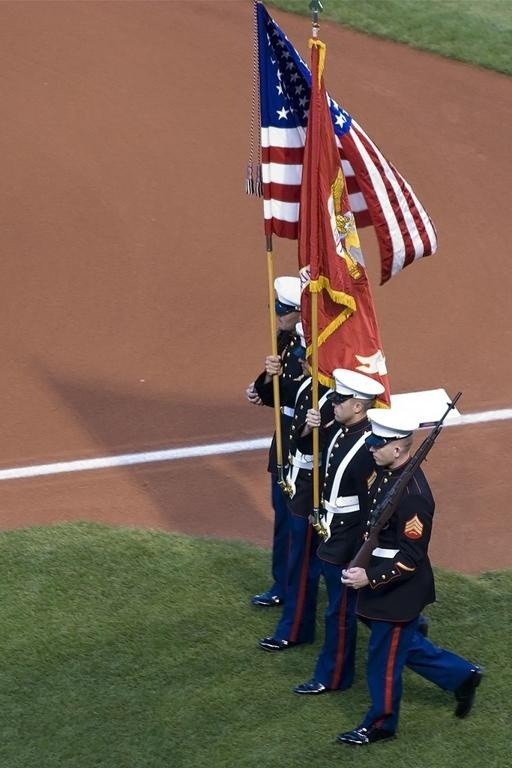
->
[274,276,302,315]
[326,368,385,404]
[365,408,418,447]
[294,322,306,358]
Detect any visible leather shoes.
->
[293,678,328,696]
[252,592,283,607]
[259,637,293,652]
[454,666,484,719]
[337,725,396,745]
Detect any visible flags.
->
[296,37,391,413]
[245,0,439,287]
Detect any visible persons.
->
[292,369,428,694]
[244,276,301,611]
[256,325,336,652]
[335,408,485,746]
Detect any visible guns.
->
[343,391,462,579]
